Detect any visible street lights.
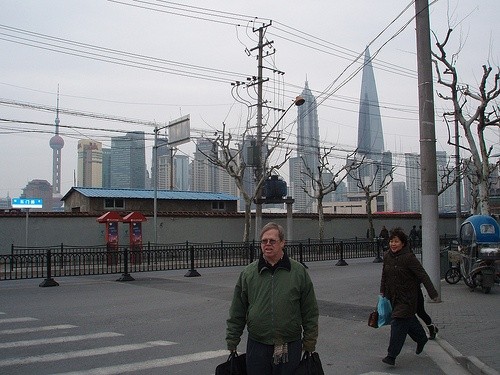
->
[254,96,305,260]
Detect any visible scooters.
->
[445,214,500,294]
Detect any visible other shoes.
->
[384,356,396,366]
[416,335,429,354]
[427,322,439,340]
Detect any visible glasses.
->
[260,238,282,244]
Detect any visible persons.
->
[416,225,422,247]
[416,286,438,340]
[380,230,440,366]
[379,225,389,252]
[225,222,320,375]
[409,225,417,250]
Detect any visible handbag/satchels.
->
[216,353,247,375]
[377,295,391,327]
[292,352,325,375]
[368,312,378,328]
